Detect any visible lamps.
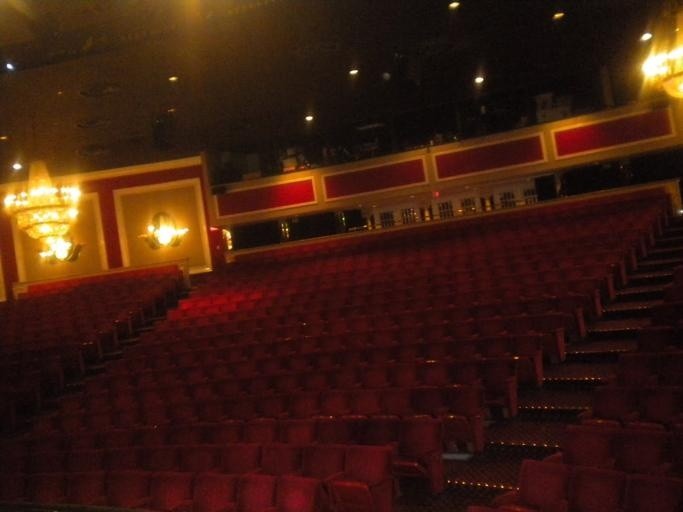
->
[635,0,683,104]
[3,165,83,264]
